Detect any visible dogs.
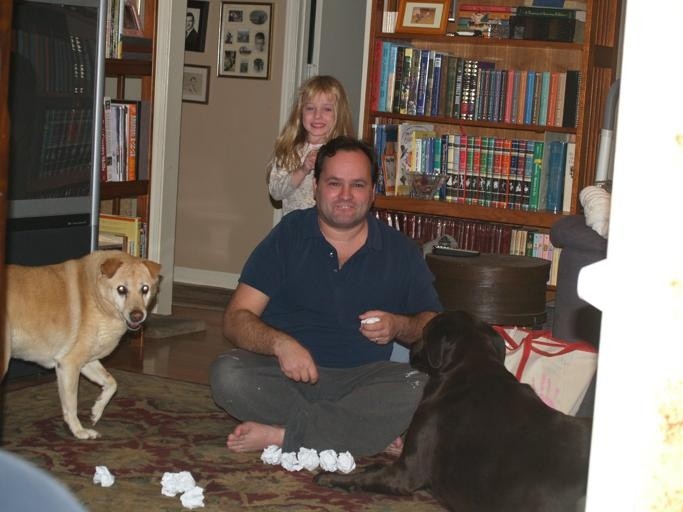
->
[1,246,162,443]
[313,311,596,511]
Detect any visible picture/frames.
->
[394,0,450,36]
[184,0,210,55]
[119,4,144,38]
[181,63,212,107]
[216,0,273,82]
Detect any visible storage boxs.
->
[508,12,575,44]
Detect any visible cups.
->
[477,21,497,39]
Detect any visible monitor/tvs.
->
[7,0,106,230]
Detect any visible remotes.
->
[432,245,480,257]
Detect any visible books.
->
[370,116,576,213]
[371,208,563,286]
[10,0,150,260]
[369,38,582,129]
[454,1,576,43]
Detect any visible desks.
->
[422,248,552,328]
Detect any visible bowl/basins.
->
[406,174,449,200]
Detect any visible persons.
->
[255,32,265,51]
[184,12,200,51]
[209,135,445,462]
[264,74,358,218]
[188,76,198,94]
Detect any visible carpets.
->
[0,364,457,510]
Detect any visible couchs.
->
[546,182,611,351]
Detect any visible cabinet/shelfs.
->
[364,0,622,291]
[97,0,160,363]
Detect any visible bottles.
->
[445,18,456,37]
